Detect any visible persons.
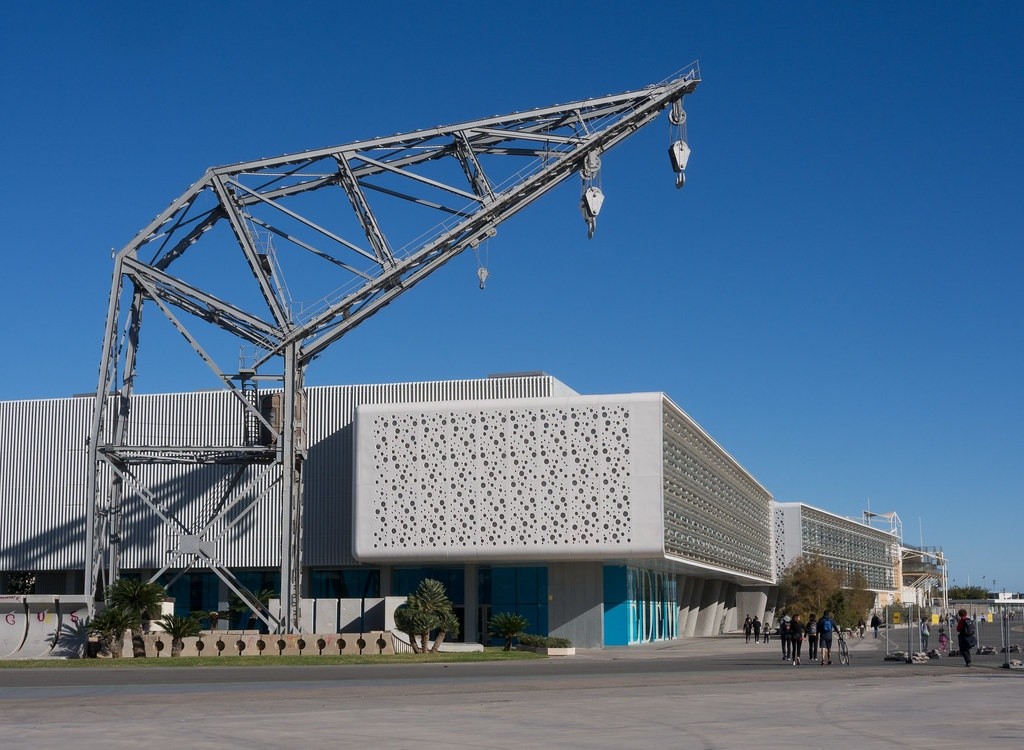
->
[1003,610,1016,625]
[957,609,977,668]
[921,616,931,652]
[938,626,948,651]
[743,614,772,644]
[858,616,866,638]
[939,612,987,628]
[780,610,840,666]
[871,613,882,639]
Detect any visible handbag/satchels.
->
[923,625,929,636]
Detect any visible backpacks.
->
[821,618,831,632]
[784,622,791,633]
[961,617,975,636]
[809,621,817,636]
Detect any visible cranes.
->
[78,59,710,658]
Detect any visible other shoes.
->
[828,660,833,664]
[783,654,788,660]
[788,659,791,661]
[810,658,813,660]
[965,660,973,667]
[797,657,801,665]
[821,660,826,664]
[792,661,796,666]
[814,657,818,660]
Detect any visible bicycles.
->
[832,630,853,666]
